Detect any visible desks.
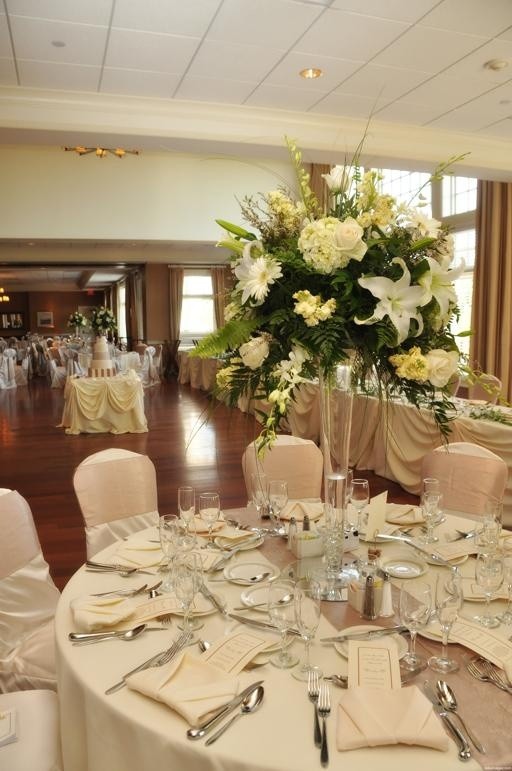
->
[176,345,511,496]
[55,374,150,435]
[176,346,512,531]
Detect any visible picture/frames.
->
[1,311,25,330]
[37,311,54,328]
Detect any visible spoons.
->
[76,624,146,649]
[203,687,265,747]
[435,679,486,756]
[86,569,138,578]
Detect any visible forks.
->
[315,684,332,768]
[93,581,163,598]
[306,671,323,747]
[103,630,193,697]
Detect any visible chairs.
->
[0,689,61,771]
[0,488,63,693]
[419,441,508,524]
[241,433,324,504]
[0,331,162,389]
[72,446,160,562]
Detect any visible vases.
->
[75,326,80,339]
[306,349,363,602]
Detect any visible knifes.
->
[186,681,265,740]
[68,628,167,643]
[85,561,155,576]
[421,680,473,761]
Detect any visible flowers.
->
[66,311,87,327]
[182,80,512,457]
[88,306,118,332]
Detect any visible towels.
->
[386,503,428,524]
[128,651,244,727]
[333,684,447,754]
[70,593,134,636]
[277,500,324,521]
[88,544,164,572]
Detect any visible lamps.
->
[0,287,9,303]
[61,145,141,158]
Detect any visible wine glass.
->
[157,468,511,697]
